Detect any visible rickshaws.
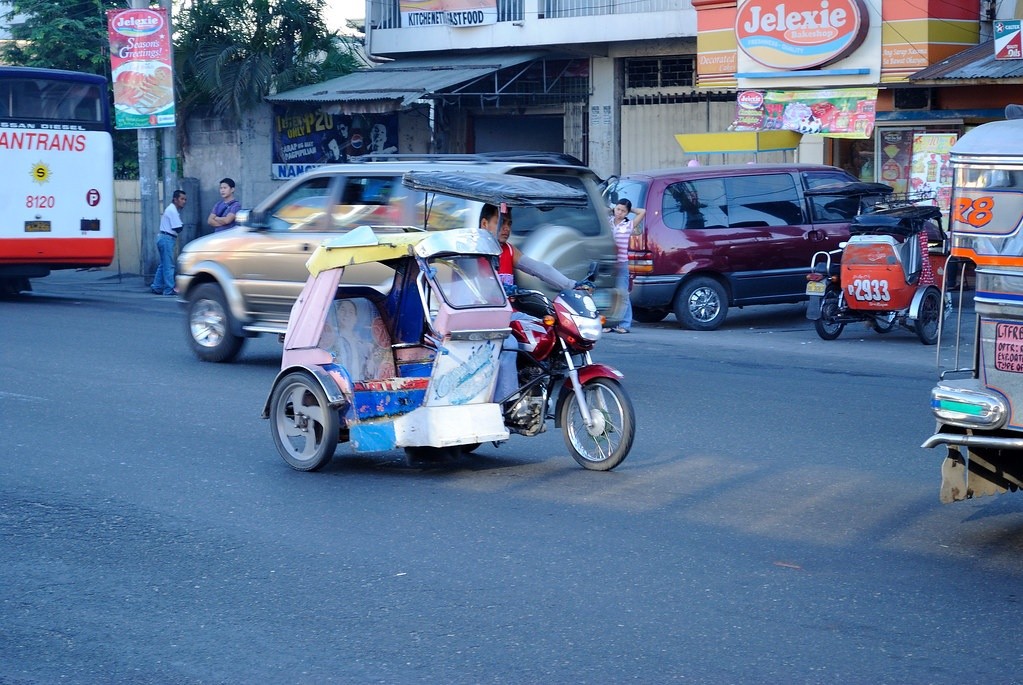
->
[263,170,638,472]
[806,192,963,344]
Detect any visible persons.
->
[479,203,593,418]
[602,199,647,334]
[207,179,241,232]
[335,118,360,146]
[363,119,398,161]
[149,190,188,296]
[321,138,348,164]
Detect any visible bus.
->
[0,66,115,299]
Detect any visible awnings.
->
[262,46,587,103]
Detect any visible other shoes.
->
[163,288,179,295]
[151,284,162,294]
[492,413,505,448]
[617,328,627,333]
[602,327,615,333]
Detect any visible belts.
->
[161,231,177,238]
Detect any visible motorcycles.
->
[922,104,1023,502]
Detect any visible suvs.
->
[603,162,949,331]
[173,153,618,363]
